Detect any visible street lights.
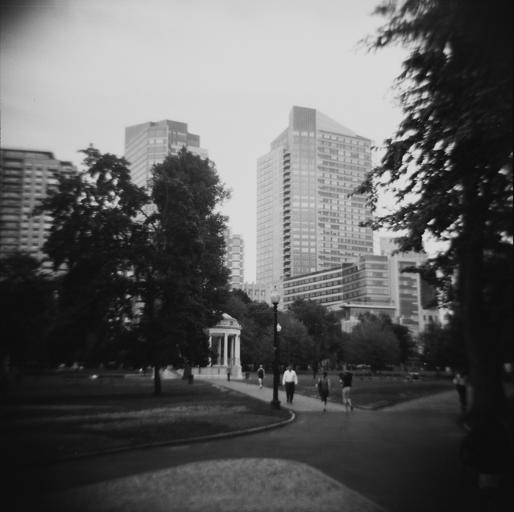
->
[268,290,282,408]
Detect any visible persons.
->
[282,365,298,403]
[338,366,353,411]
[257,364,265,388]
[316,372,330,411]
